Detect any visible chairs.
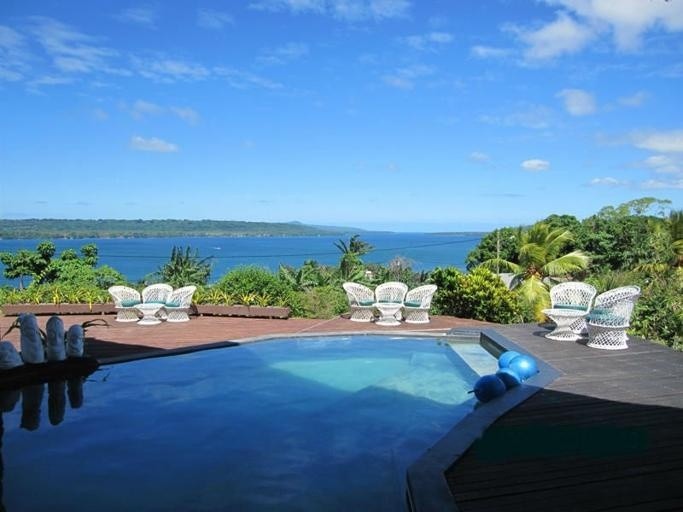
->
[107,281,196,324]
[541,280,641,350]
[343,281,438,326]
